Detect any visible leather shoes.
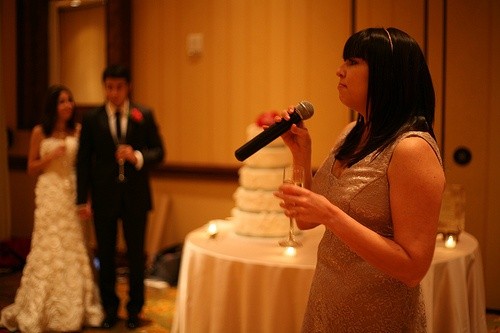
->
[127,314,153,330]
[102,315,121,330]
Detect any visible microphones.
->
[235,100,314,162]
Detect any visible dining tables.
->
[170,218,487,333]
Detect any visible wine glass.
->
[279,165,305,247]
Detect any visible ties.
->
[115,110,122,143]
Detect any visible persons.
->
[262,27,445,333]
[0,85,105,332]
[75,64,165,330]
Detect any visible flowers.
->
[129,107,144,122]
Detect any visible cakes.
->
[230,111,301,237]
